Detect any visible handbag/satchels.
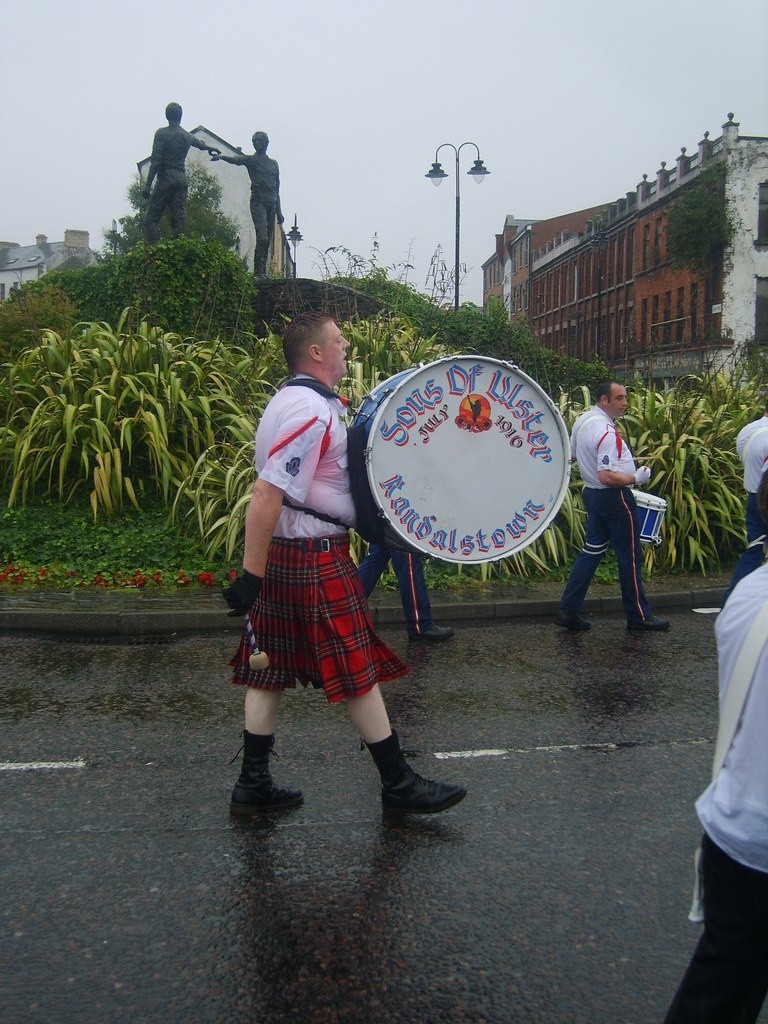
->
[688,847,709,923]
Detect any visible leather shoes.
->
[627,615,670,631]
[412,626,453,640]
[555,607,591,631]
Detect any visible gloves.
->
[220,569,263,617]
[634,466,651,485]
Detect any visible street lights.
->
[424,142,491,310]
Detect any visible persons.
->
[142,103,222,243]
[664,409,768,1024]
[221,310,467,813]
[212,134,284,281]
[356,540,456,641]
[551,380,671,631]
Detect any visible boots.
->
[360,728,467,817]
[230,730,304,815]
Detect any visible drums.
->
[345,356,575,569]
[629,485,669,547]
